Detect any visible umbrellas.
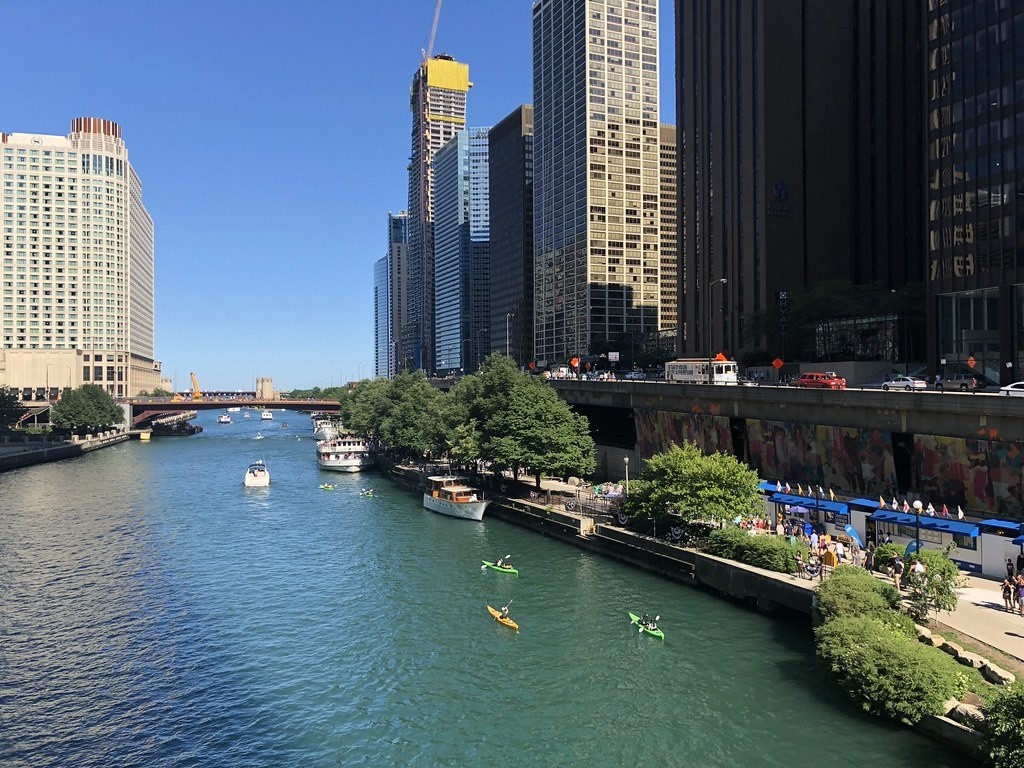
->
[788,505,809,518]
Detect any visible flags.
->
[879,496,964,520]
[776,481,834,501]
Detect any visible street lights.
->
[625,314,634,370]
[405,354,413,371]
[506,313,515,356]
[461,338,471,375]
[477,329,487,365]
[577,306,592,380]
[891,289,909,376]
[623,454,630,498]
[709,278,727,385]
[46,364,53,401]
[66,365,71,388]
[912,499,922,556]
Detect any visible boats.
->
[310,413,379,473]
[320,483,334,491]
[628,612,665,639]
[260,410,273,419]
[244,455,271,488]
[481,559,518,574]
[486,604,518,630]
[362,488,374,497]
[280,421,289,430]
[422,474,492,521]
[242,411,250,418]
[217,410,232,423]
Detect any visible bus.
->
[665,359,738,385]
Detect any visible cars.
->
[881,377,927,391]
[999,381,1024,397]
[796,373,846,390]
[625,371,646,380]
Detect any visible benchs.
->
[543,477,560,480]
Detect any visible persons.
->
[849,528,924,591]
[925,376,930,387]
[551,370,615,381]
[594,482,623,498]
[750,370,769,382]
[547,489,551,497]
[1001,554,1024,617]
[795,550,803,578]
[734,513,844,564]
[935,373,941,380]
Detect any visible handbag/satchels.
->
[1003,593,1009,599]
[768,520,772,525]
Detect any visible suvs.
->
[934,374,984,393]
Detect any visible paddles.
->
[638,625,646,633]
[481,564,487,569]
[492,599,514,621]
[630,621,637,625]
[655,615,661,621]
[502,554,511,560]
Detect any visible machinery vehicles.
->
[189,372,203,401]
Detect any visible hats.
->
[1005,576,1010,582]
[502,607,508,614]
[644,614,649,617]
[892,552,897,556]
[813,530,815,532]
